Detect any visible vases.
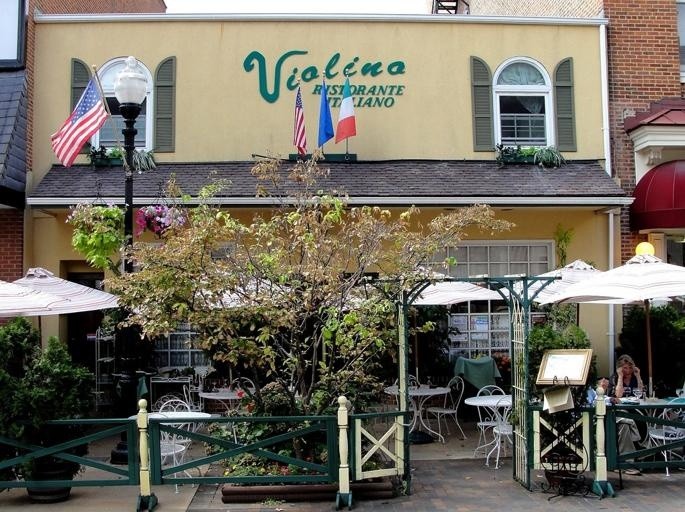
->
[91,218,120,232]
[146,216,171,239]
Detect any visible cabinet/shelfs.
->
[96,326,115,412]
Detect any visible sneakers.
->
[613,467,641,475]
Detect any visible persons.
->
[571,377,642,476]
[608,354,650,451]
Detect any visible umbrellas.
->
[498,258,604,327]
[326,264,504,382]
[12,267,126,356]
[1,280,72,313]
[538,254,685,397]
[132,268,296,385]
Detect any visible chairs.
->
[586,395,685,477]
[384,375,512,471]
[129,371,257,494]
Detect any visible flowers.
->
[135,205,185,240]
[65,203,125,269]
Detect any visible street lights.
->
[635,239,658,400]
[113,54,150,464]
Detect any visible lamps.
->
[635,242,655,256]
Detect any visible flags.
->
[317,79,335,148]
[292,85,307,158]
[51,74,110,170]
[335,78,356,143]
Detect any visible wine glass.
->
[624,385,648,400]
[426,376,432,386]
[212,378,228,393]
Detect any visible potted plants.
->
[12,336,96,504]
[86,141,157,173]
[496,142,567,168]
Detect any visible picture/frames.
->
[535,348,593,386]
